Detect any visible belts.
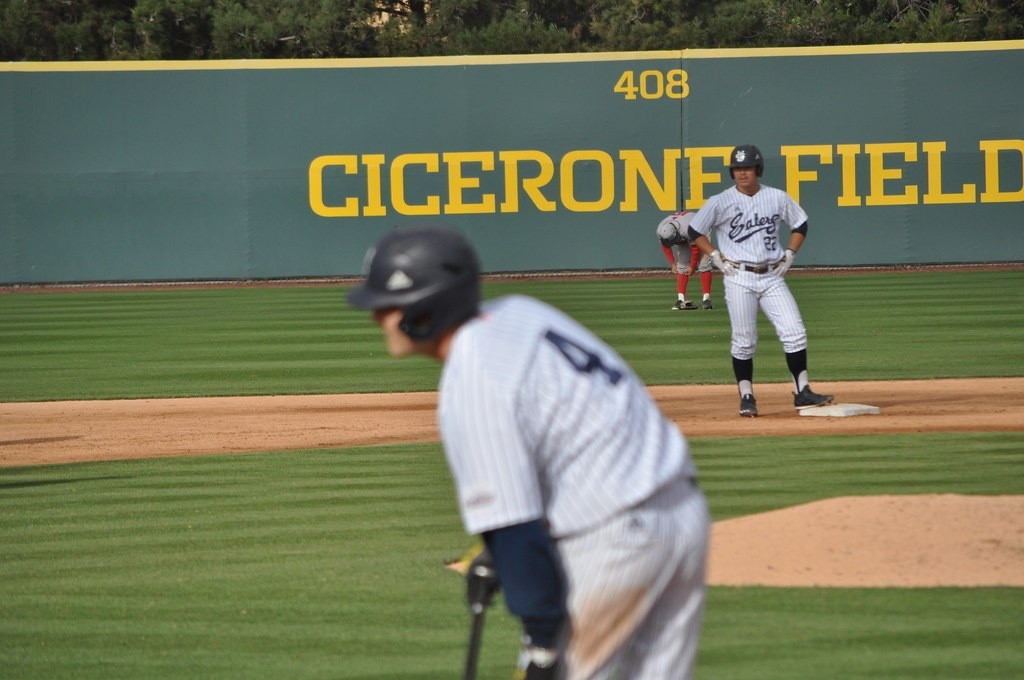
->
[733,262,778,273]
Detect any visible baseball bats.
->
[463,564,492,680]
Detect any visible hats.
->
[657,222,677,242]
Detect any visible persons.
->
[656,211,715,310]
[346,224,708,680]
[687,145,833,417]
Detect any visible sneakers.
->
[702,299,712,310]
[792,385,833,410]
[739,393,757,416]
[672,300,685,311]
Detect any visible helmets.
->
[729,145,763,180]
[346,224,481,340]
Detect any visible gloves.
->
[709,250,738,277]
[517,643,565,680]
[772,248,796,277]
[467,551,498,614]
